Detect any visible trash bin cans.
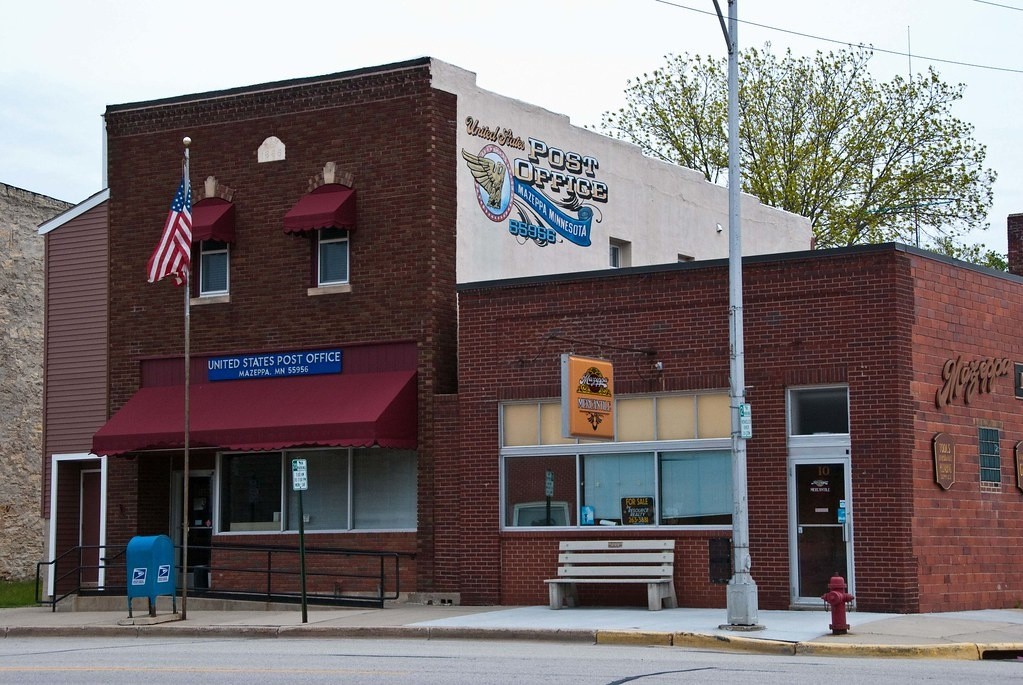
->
[124,536,177,616]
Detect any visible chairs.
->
[544,540,678,611]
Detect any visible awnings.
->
[87,370,420,456]
[191,203,237,244]
[283,189,355,233]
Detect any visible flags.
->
[144,168,194,289]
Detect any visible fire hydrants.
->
[821,572,855,635]
